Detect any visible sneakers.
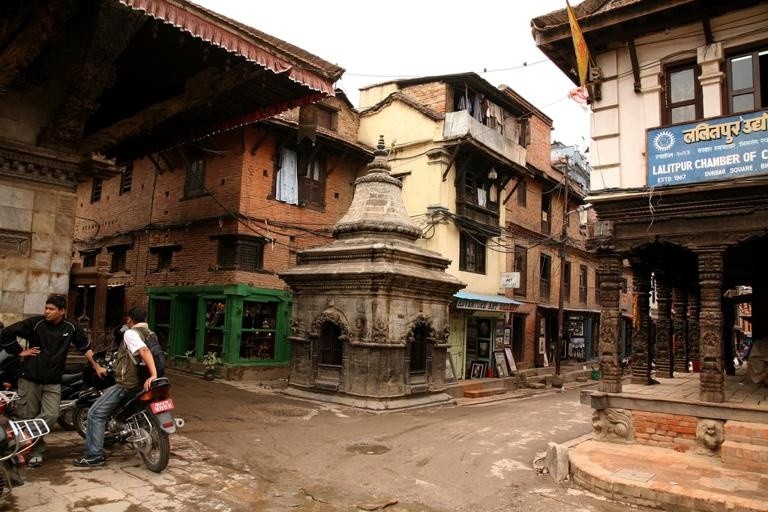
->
[72,453,105,467]
[85,448,114,464]
[28,455,44,467]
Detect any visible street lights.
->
[555,200,593,374]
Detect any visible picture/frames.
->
[538,315,546,356]
[466,317,517,379]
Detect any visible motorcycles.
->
[0,323,188,499]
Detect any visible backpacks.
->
[137,327,165,385]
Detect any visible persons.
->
[72,306,159,467]
[742,341,750,367]
[0,296,108,467]
[113,312,130,348]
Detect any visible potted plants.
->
[202,351,222,379]
